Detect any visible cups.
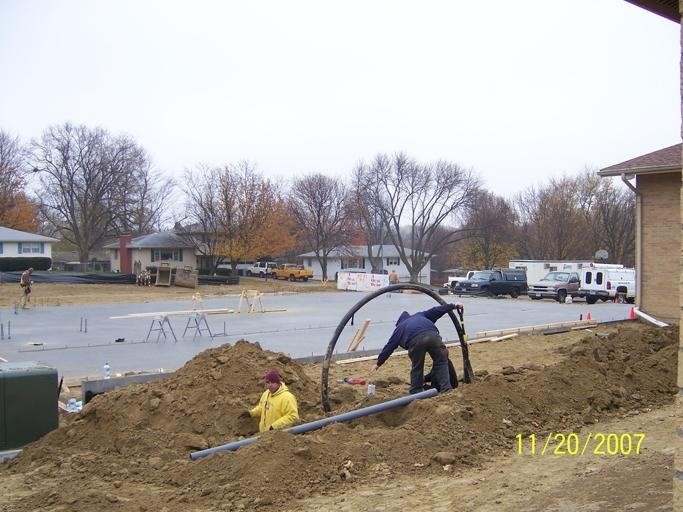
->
[367,384,375,395]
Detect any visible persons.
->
[389,270,397,284]
[369,303,464,394]
[20,268,34,302]
[238,371,300,432]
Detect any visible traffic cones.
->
[586,308,590,320]
[630,307,634,318]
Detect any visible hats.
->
[265,368,280,383]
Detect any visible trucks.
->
[577,268,635,304]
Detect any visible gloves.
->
[238,411,250,419]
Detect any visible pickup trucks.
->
[528,270,581,303]
[272,264,313,282]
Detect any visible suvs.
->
[453,270,528,298]
[247,262,278,278]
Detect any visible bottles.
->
[103,363,111,380]
[69,397,83,411]
